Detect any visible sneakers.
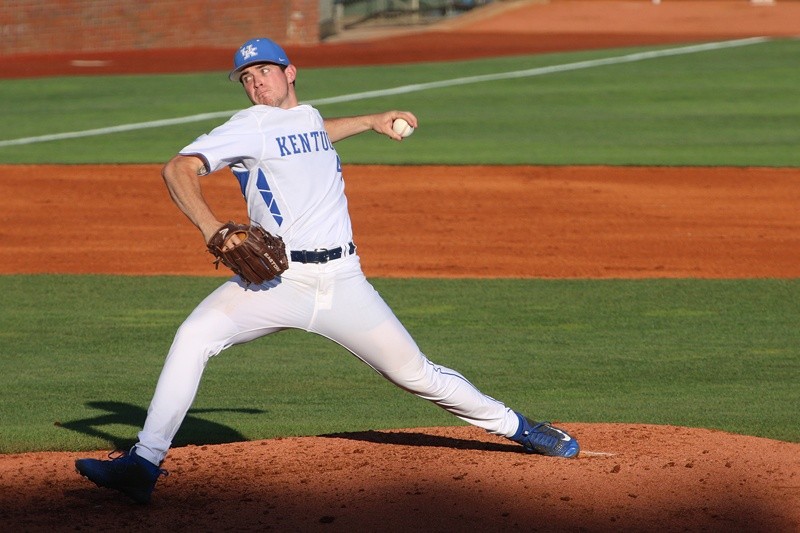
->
[509,412,580,459]
[75,444,168,502]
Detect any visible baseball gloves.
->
[206,222,289,286]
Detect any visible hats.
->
[227,37,291,82]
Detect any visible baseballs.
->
[392,119,415,137]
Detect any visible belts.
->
[290,243,356,264]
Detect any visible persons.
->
[75,38,579,504]
